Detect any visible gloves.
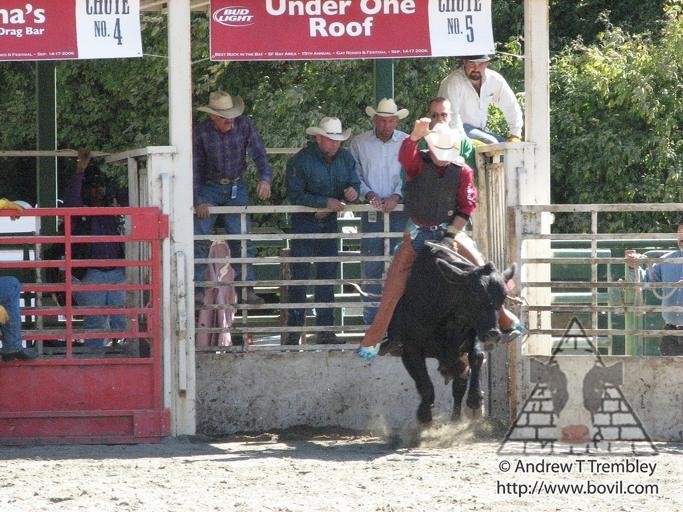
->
[0,306,10,324]
[5,202,22,220]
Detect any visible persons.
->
[351,95,412,326]
[436,57,523,143]
[400,97,478,203]
[283,117,360,346]
[626,216,683,355]
[61,148,128,346]
[356,117,524,359]
[190,90,274,306]
[0,197,34,361]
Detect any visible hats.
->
[461,55,490,63]
[196,90,244,117]
[364,97,409,120]
[306,117,352,140]
[423,123,461,162]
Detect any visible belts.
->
[417,225,443,231]
[209,176,241,184]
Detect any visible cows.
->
[384,238,518,430]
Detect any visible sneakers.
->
[238,290,264,304]
[3,348,39,360]
[318,336,345,344]
